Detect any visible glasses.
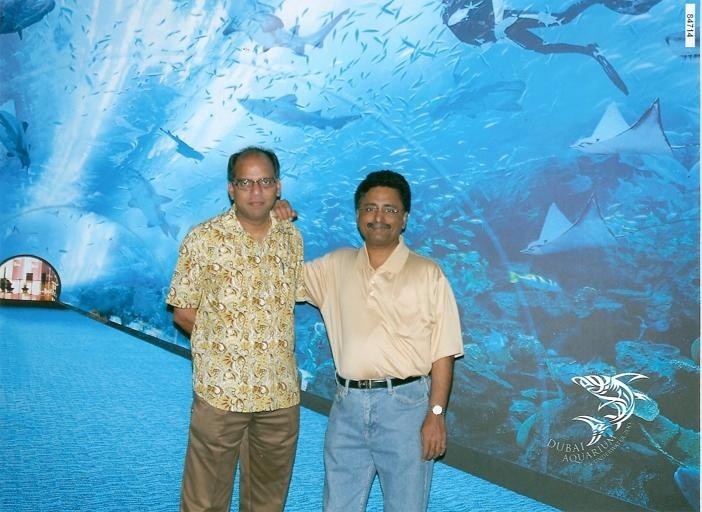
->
[357,206,406,216]
[232,177,278,191]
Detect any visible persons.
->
[165,145,308,511]
[269,170,464,511]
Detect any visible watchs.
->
[427,404,448,416]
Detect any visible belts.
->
[335,373,422,390]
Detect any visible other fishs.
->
[0,2,700,512]
